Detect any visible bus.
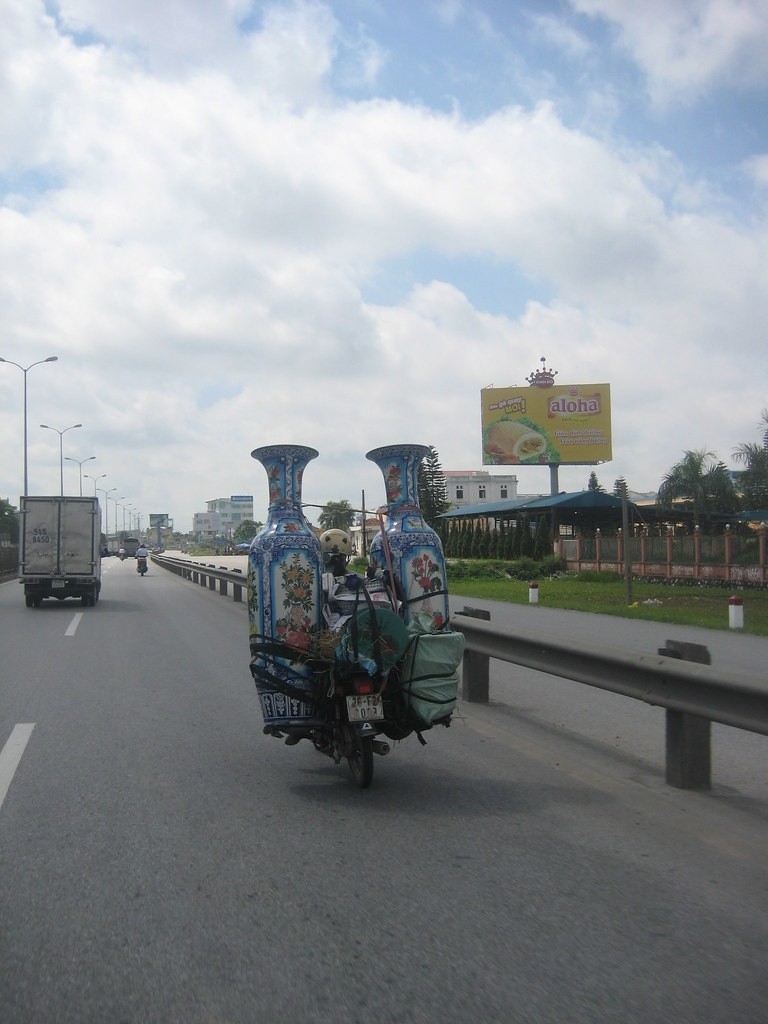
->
[123,538,141,557]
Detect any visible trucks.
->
[17,494,104,607]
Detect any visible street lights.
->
[64,456,96,497]
[116,503,132,538]
[96,488,117,536]
[84,474,106,496]
[0,355,60,495]
[108,495,126,537]
[39,422,82,496]
[124,507,136,537]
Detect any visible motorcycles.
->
[118,552,127,561]
[280,672,391,790]
[135,556,149,576]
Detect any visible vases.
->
[251,445,325,734]
[366,444,451,635]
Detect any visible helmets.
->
[140,544,145,548]
[319,528,352,556]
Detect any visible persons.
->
[285,529,353,746]
[120,547,127,559]
[135,544,149,573]
